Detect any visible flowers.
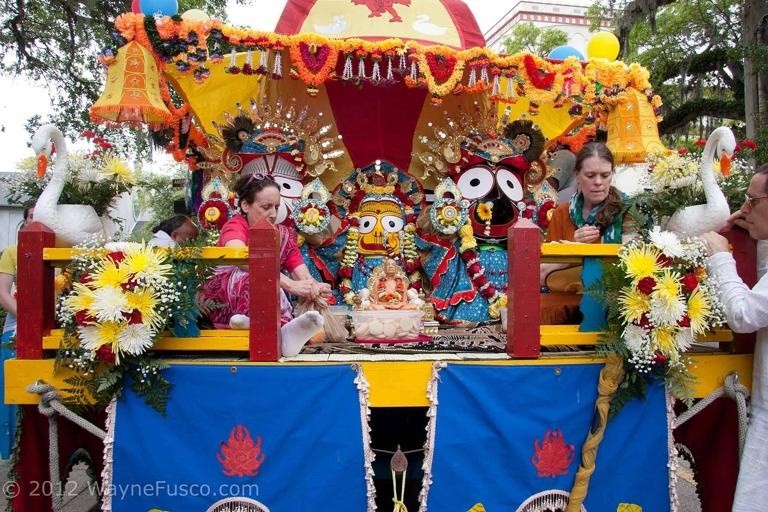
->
[576,228,730,420]
[55,234,224,417]
[1,128,160,232]
[629,141,756,228]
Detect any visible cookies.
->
[354,318,420,341]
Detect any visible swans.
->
[32,123,108,251]
[664,126,736,239]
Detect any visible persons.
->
[363,260,410,309]
[196,173,333,356]
[151,214,201,262]
[691,160,768,512]
[535,139,647,321]
[1,199,63,460]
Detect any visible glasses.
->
[246,172,275,184]
[744,193,768,209]
